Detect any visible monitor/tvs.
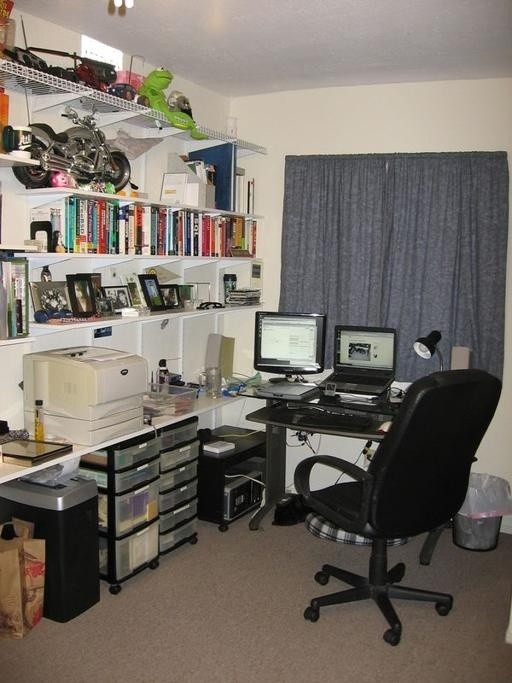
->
[254,311,326,384]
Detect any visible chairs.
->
[294,351,503,646]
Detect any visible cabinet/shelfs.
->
[0,61,266,485]
[79,428,160,593]
[155,418,201,553]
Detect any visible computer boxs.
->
[224,468,262,521]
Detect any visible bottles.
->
[155,358,170,394]
[33,399,46,442]
[0,87,11,154]
[223,272,238,304]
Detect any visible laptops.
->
[318,325,397,397]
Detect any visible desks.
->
[246,401,398,531]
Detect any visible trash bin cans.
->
[452,473,508,552]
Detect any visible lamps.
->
[113,0,134,8]
[412,331,444,371]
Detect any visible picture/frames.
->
[101,286,131,313]
[138,275,165,310]
[30,282,70,322]
[120,276,148,309]
[66,274,96,318]
[159,284,181,309]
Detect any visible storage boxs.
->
[144,384,199,418]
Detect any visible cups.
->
[0,17,16,62]
[199,367,222,400]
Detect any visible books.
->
[0,191,262,340]
[185,141,258,213]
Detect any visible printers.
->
[23,345,147,446]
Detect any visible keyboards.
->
[270,406,373,432]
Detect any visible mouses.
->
[378,421,393,432]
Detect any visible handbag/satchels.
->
[0,515,50,642]
